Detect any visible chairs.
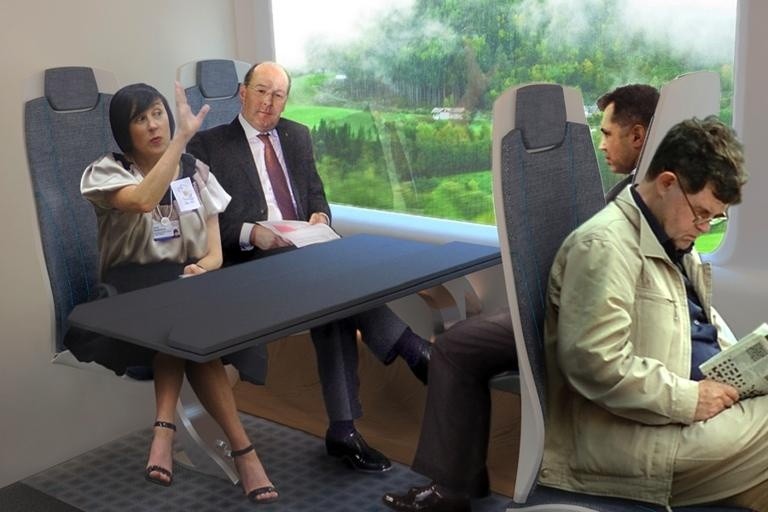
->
[178,57,255,355]
[604,71,722,235]
[490,84,697,511]
[22,66,242,483]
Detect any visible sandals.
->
[230,444,279,505]
[144,419,177,487]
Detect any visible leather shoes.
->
[324,429,393,475]
[382,482,473,511]
[410,344,436,386]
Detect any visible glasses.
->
[674,171,730,227]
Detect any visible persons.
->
[381,83,661,512]
[81,67,282,504]
[188,62,437,472]
[532,114,768,510]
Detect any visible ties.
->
[256,132,298,220]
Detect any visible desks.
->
[66,232,500,363]
